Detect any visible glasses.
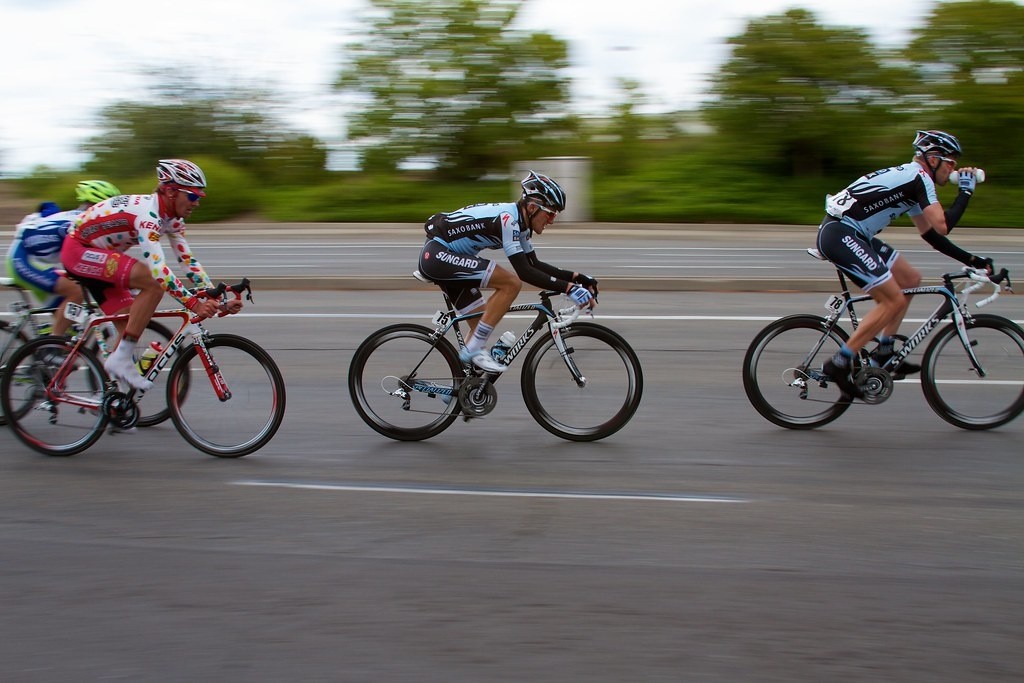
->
[925,155,959,171]
[174,185,201,201]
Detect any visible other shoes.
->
[41,351,80,371]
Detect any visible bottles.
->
[135,341,163,377]
[948,168,985,185]
[489,330,517,362]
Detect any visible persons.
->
[59,159,244,432]
[5,181,124,371]
[816,131,994,402]
[416,170,598,406]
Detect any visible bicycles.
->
[347,289,643,443]
[0,290,193,428]
[741,248,1024,430]
[0,279,286,457]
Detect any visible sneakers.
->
[105,353,154,390]
[870,347,921,375]
[822,357,864,402]
[458,346,507,375]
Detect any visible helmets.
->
[912,128,963,160]
[156,158,207,189]
[75,178,120,203]
[522,169,566,212]
